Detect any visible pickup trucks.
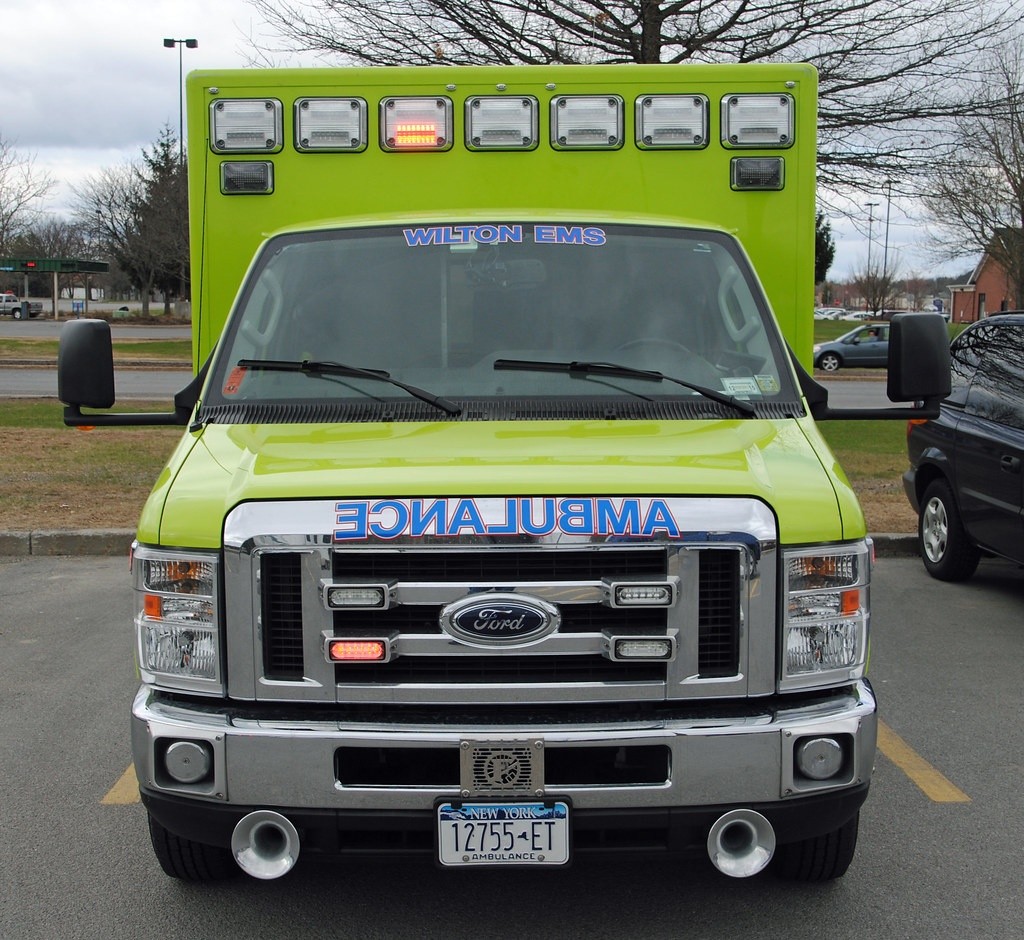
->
[0,294,43,318]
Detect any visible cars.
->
[901,307,1024,582]
[812,323,891,371]
[814,309,874,321]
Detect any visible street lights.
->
[95,209,102,259]
[863,202,881,311]
[162,37,199,316]
[880,178,901,320]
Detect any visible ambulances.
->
[58,63,953,887]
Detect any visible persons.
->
[869,329,878,342]
